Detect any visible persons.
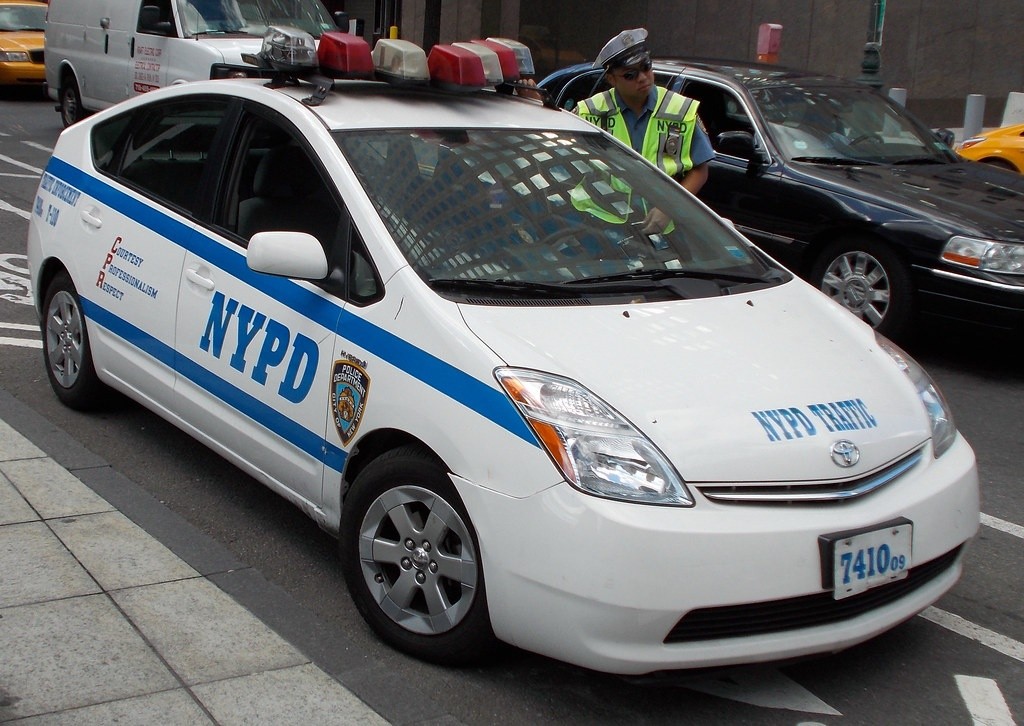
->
[515,26,714,253]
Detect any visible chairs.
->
[238,146,340,239]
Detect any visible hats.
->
[591,28,648,73]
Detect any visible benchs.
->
[129,118,270,211]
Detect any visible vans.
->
[43,0,339,131]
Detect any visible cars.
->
[0,0,49,91]
[953,123,1023,175]
[25,25,983,673]
[536,56,1024,363]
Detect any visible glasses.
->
[614,60,653,81]
